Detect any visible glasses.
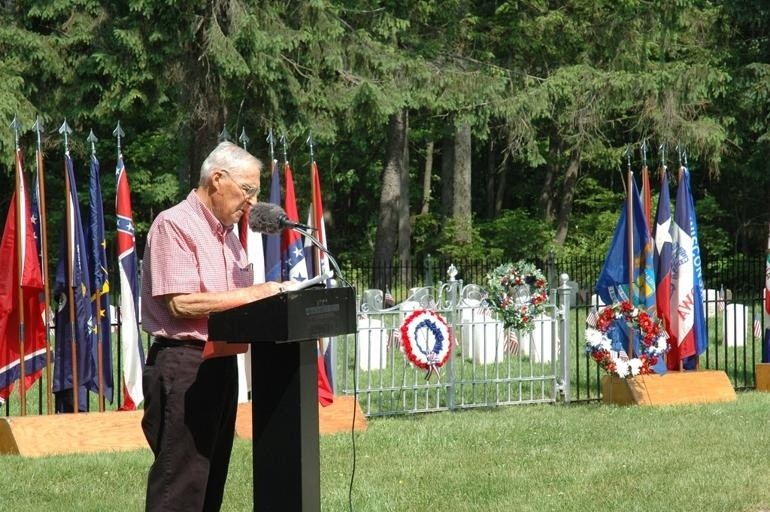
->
[220,170,260,196]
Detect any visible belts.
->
[153,335,207,349]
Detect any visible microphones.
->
[248,201,317,235]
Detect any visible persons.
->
[141,142,289,512]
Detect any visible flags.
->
[236,158,334,408]
[0,147,147,413]
[591,165,708,378]
[762,231,770,363]
